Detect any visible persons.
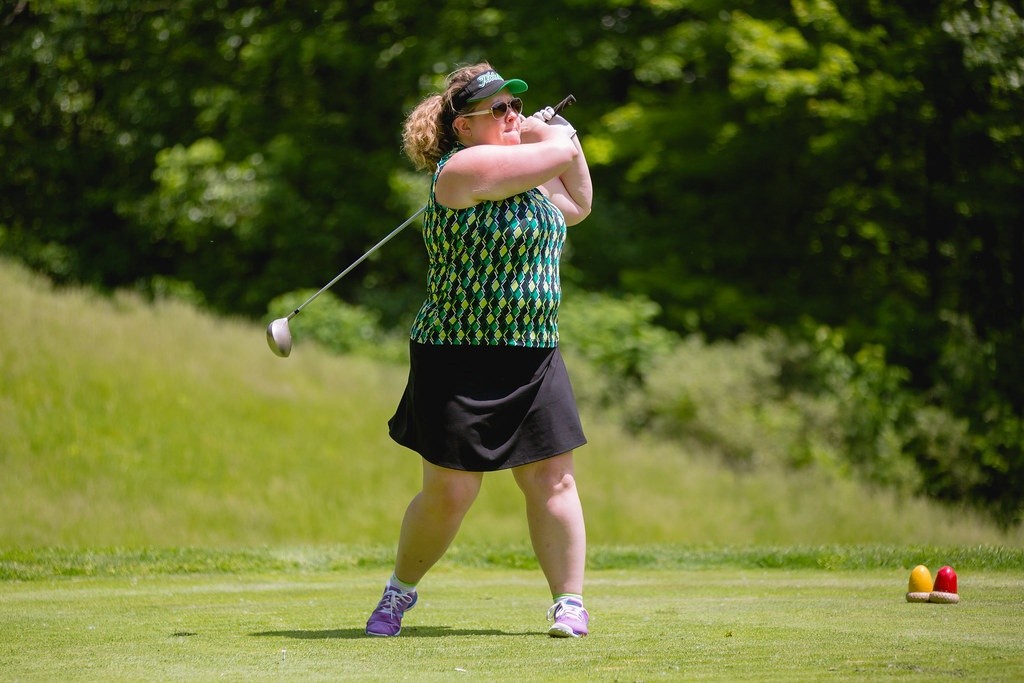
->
[365,66,597,640]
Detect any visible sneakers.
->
[365,580,418,637]
[546,598,588,637]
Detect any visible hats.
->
[440,69,528,125]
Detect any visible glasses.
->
[457,97,523,120]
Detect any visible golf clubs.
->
[264,92,579,359]
